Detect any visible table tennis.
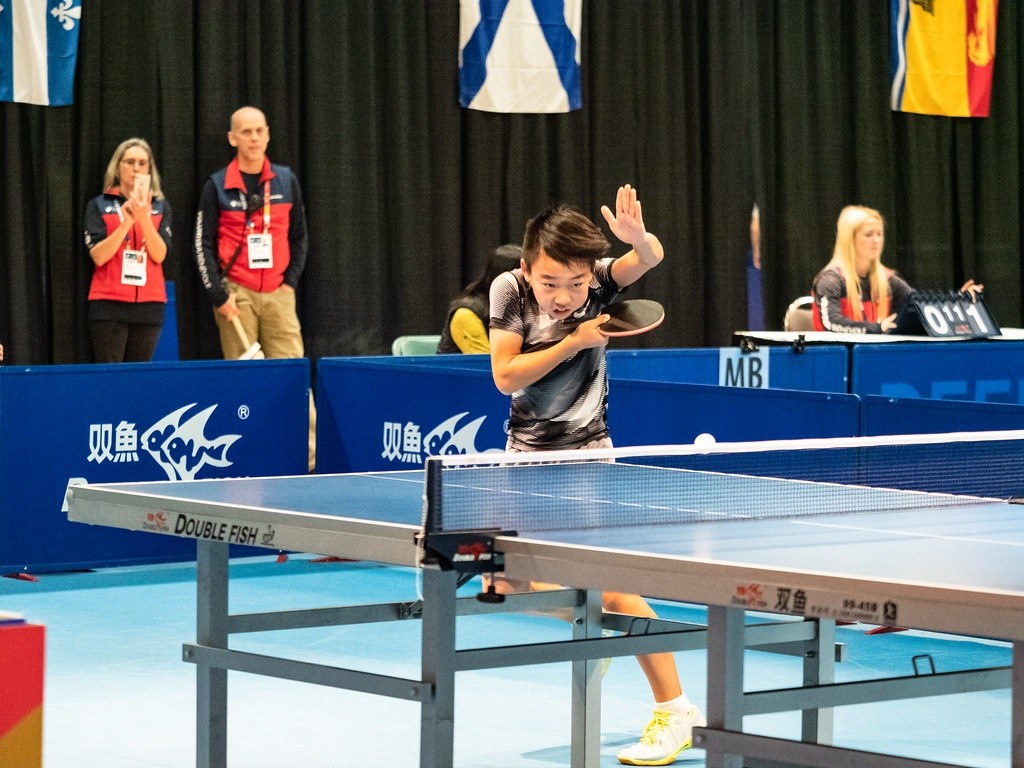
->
[693,432,716,455]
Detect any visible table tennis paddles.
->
[557,298,665,338]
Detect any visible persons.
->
[813,206,984,333]
[482,184,707,765]
[437,245,524,354]
[84,138,171,362]
[194,107,316,472]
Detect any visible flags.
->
[890,0,997,117]
[459,0,583,114]
[0,0,82,105]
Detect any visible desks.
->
[734,327,1024,346]
[66,459,1024,768]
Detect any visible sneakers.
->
[616,705,707,766]
[596,609,610,678]
